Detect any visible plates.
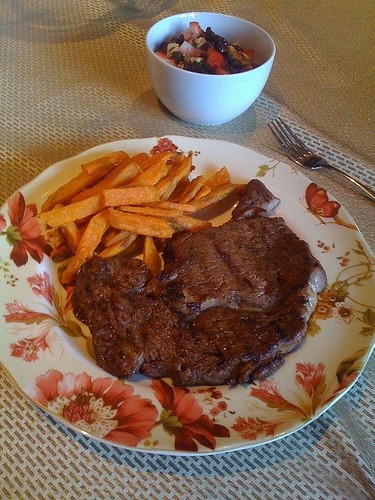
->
[0,136,375,457]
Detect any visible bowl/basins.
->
[143,10,275,128]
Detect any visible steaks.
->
[72,179,328,389]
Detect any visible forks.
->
[267,118,375,202]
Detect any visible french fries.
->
[36,151,249,285]
